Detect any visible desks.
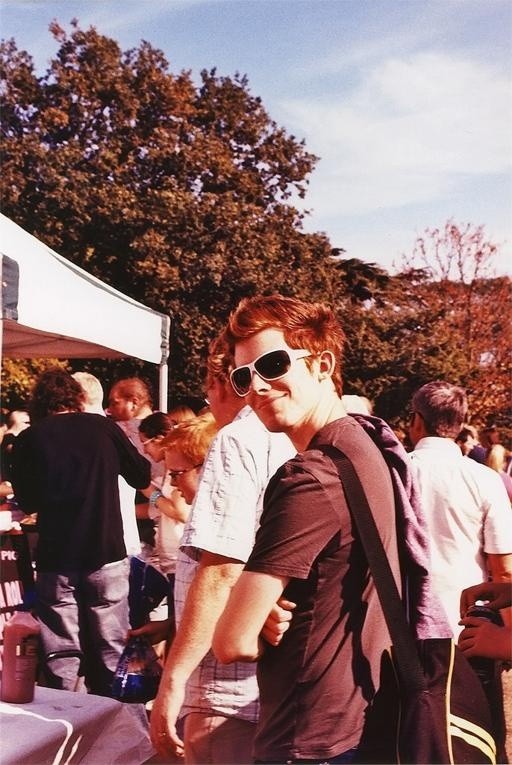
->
[0,680,159,764]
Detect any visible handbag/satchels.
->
[357,641,497,763]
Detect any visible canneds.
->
[463,605,497,684]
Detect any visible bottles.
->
[1,604,42,703]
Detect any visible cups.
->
[464,605,502,682]
[2,480,15,500]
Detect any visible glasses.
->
[169,464,203,479]
[230,347,321,397]
